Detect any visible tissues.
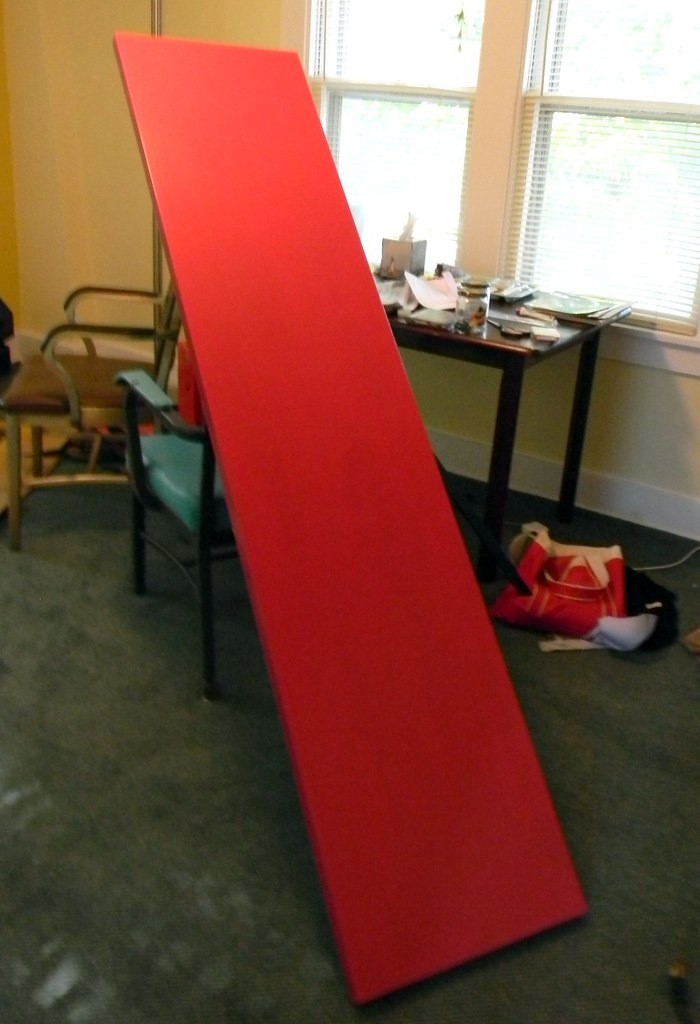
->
[380,212,427,280]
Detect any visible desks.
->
[389,300,632,584]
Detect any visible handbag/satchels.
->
[489,522,626,637]
[625,566,679,652]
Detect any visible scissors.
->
[487,319,531,340]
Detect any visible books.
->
[524,291,631,326]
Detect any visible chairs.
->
[112,368,238,703]
[0,280,183,552]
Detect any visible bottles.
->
[454,277,491,334]
